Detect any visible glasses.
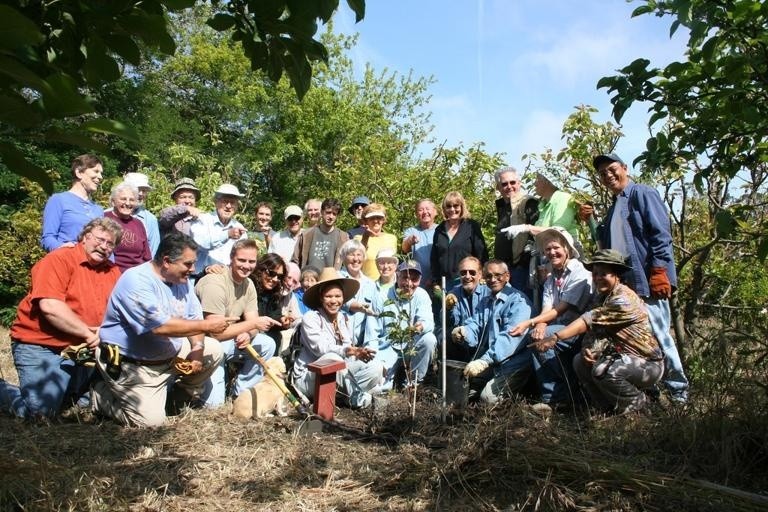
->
[445,204,460,209]
[500,179,518,188]
[90,230,116,249]
[459,270,477,276]
[265,268,284,280]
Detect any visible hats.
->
[374,248,399,265]
[535,225,581,260]
[399,260,423,275]
[283,205,303,220]
[170,177,201,202]
[536,160,571,192]
[123,172,153,192]
[581,248,632,274]
[213,183,245,199]
[348,196,371,217]
[364,211,385,219]
[302,267,360,311]
[592,153,624,172]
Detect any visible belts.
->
[118,354,173,367]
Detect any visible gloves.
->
[462,359,489,378]
[648,267,672,301]
[445,293,457,310]
[500,224,526,240]
[451,325,466,344]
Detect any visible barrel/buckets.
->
[437,360,472,410]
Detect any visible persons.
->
[39,154,115,265]
[526,249,665,418]
[579,153,690,410]
[0,215,124,426]
